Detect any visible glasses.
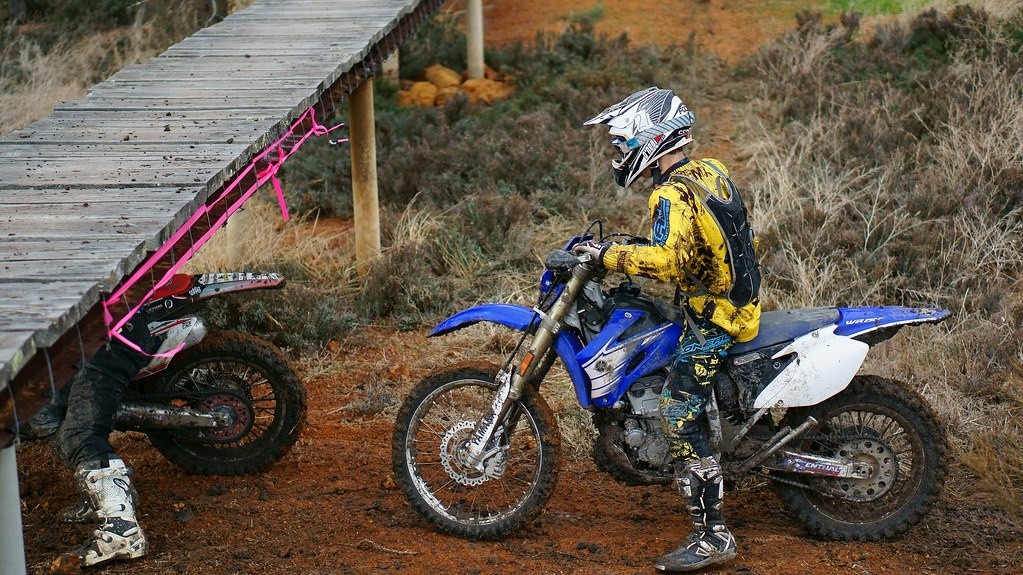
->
[609,137,629,159]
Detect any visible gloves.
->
[572,240,611,266]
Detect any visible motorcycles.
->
[16,271,310,478]
[391,216,950,542]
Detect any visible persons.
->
[26,312,153,565]
[572,86,762,573]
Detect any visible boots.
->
[654,469,737,571]
[74,454,148,568]
[57,478,142,523]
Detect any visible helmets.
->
[583,87,694,189]
